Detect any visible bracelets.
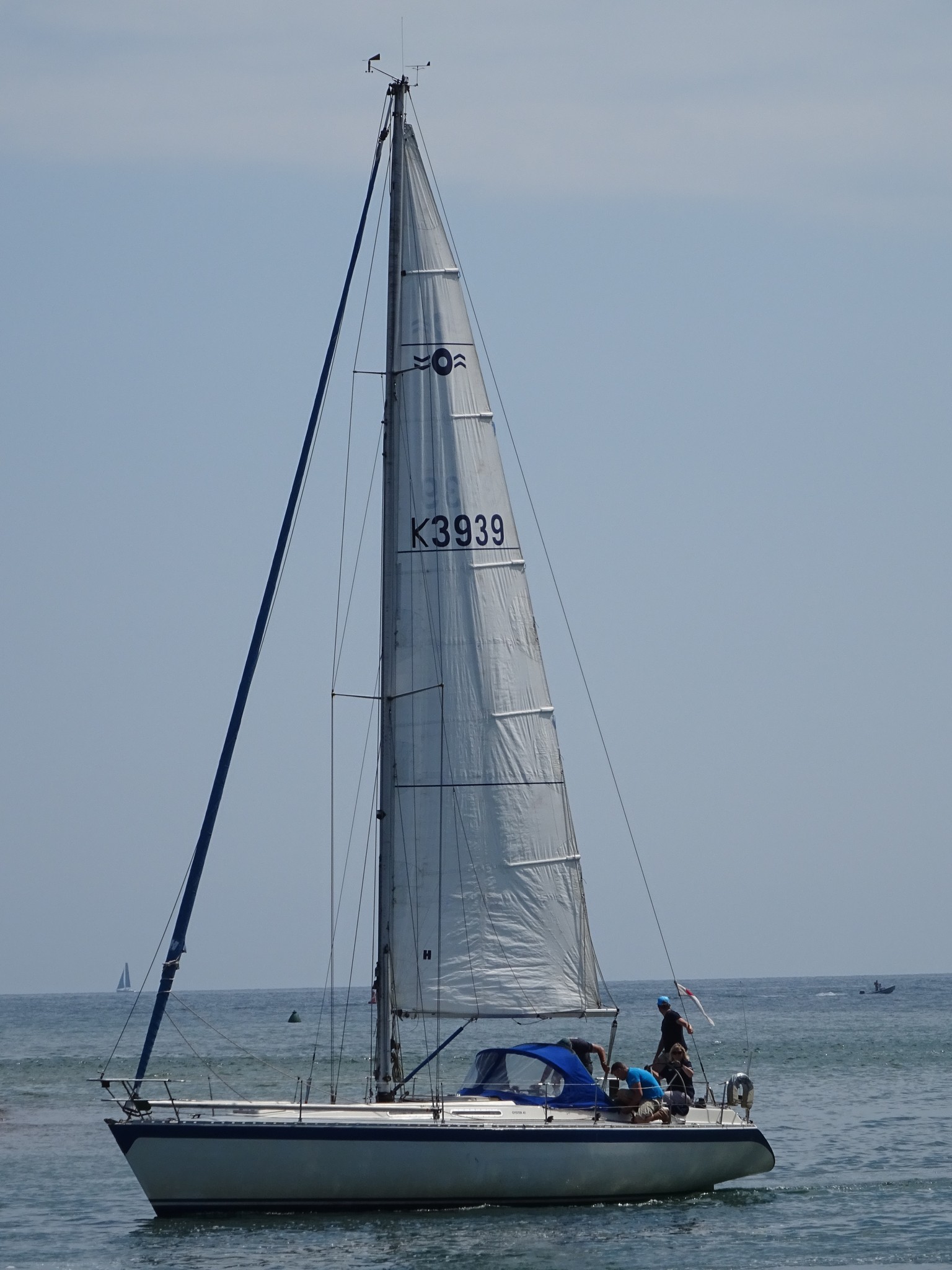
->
[679,1064,683,1068]
[601,1061,606,1065]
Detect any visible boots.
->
[654,1107,671,1125]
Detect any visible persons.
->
[645,1042,695,1106]
[651,996,694,1088]
[531,1037,611,1098]
[874,980,879,991]
[610,1062,670,1125]
[878,983,882,989]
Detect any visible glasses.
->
[672,1051,682,1055]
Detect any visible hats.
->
[657,996,671,1006]
[560,1038,570,1047]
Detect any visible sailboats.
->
[92,46,784,1212]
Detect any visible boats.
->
[858,985,896,996]
